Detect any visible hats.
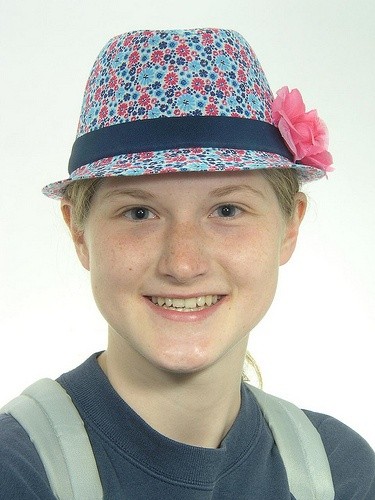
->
[42,28,335,202]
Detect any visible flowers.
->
[271,86,336,172]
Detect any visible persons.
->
[0,26,375,499]
